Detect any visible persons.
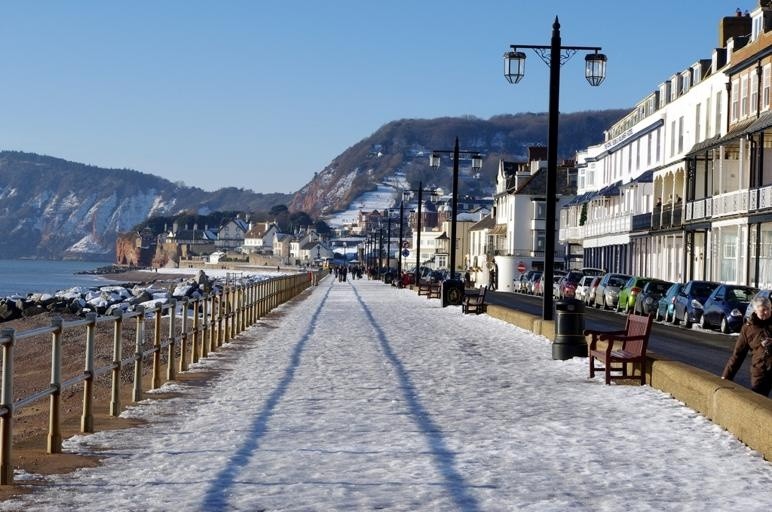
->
[721,296,772,396]
[333,264,496,291]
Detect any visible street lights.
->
[503,14,605,321]
[366,200,415,287]
[401,184,439,287]
[430,136,483,280]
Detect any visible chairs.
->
[462,284,487,315]
[418,277,432,295]
[583,310,652,386]
[426,280,443,299]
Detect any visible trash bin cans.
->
[552,298,588,360]
[406,273,415,290]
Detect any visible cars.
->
[671,281,720,328]
[701,285,762,334]
[407,266,461,281]
[743,289,771,333]
[513,268,686,321]
